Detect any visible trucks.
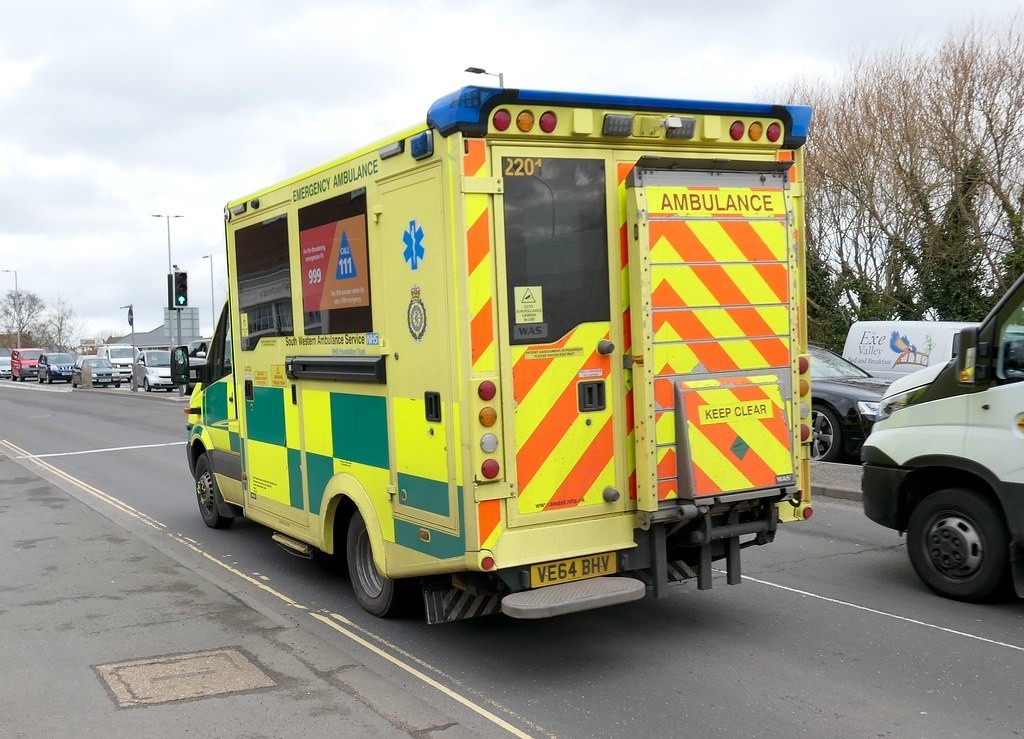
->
[97,343,140,382]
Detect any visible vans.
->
[0,348,47,382]
[860,272,1024,602]
[183,340,232,394]
[843,322,1022,382]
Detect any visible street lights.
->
[202,254,215,332]
[2,270,20,349]
[466,68,503,86]
[152,214,184,274]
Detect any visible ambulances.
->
[170,86,813,624]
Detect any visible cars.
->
[130,351,179,392]
[38,353,76,383]
[72,355,121,388]
[807,344,890,461]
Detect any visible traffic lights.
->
[175,272,187,306]
